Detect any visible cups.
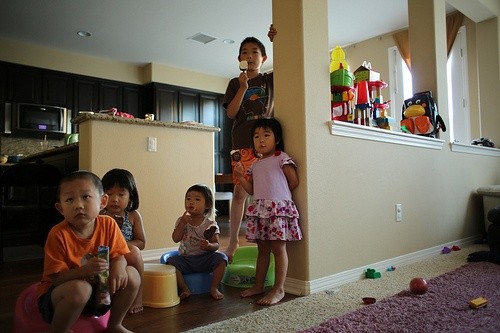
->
[41,141,48,151]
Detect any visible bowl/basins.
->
[7,155,23,163]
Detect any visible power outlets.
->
[395,203,402,223]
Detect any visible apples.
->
[410,278,427,294]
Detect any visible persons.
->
[97,168,146,314]
[220,36,276,264]
[166,184,228,300]
[231,116,304,306]
[36,171,143,333]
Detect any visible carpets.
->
[182,239,500,333]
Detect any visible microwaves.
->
[12,104,67,138]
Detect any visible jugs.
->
[66,134,79,145]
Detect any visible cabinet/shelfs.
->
[0,60,234,247]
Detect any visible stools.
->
[222,244,275,288]
[141,263,181,308]
[11,282,110,333]
[473,184,500,243]
